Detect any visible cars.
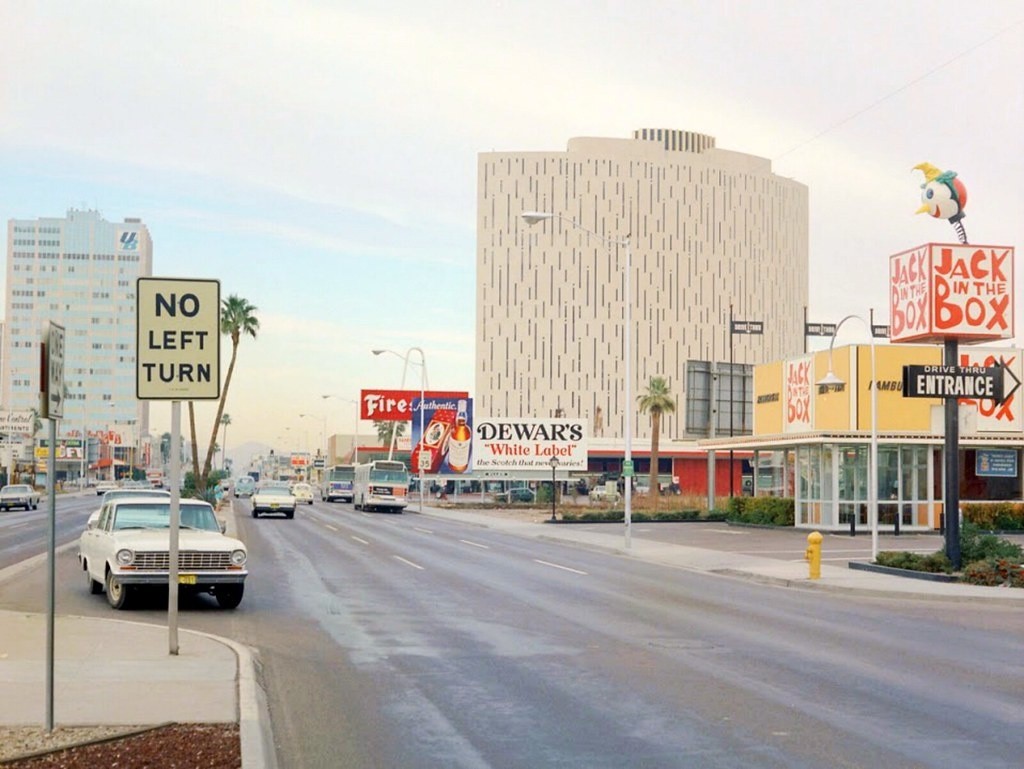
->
[77,490,249,610]
[291,484,314,505]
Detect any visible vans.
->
[234,476,255,499]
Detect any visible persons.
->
[887,489,912,524]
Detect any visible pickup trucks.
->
[0,484,41,511]
[95,481,119,496]
[250,485,297,519]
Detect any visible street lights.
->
[371,347,428,515]
[322,393,358,462]
[550,455,559,519]
[815,315,879,563]
[522,211,633,546]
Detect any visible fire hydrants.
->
[804,530,824,580]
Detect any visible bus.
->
[321,466,356,503]
[351,460,409,514]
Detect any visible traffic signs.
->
[46,318,65,421]
